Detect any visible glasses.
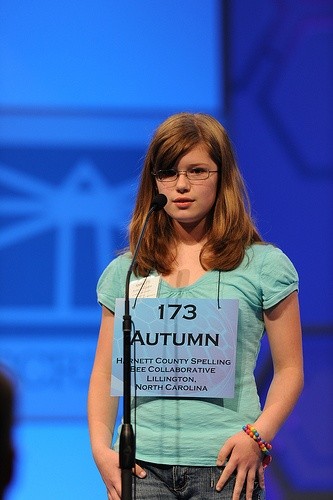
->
[150,167,219,182]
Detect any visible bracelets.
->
[242,424,272,456]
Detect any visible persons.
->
[88,111,303,499]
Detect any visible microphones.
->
[123,194,168,331]
